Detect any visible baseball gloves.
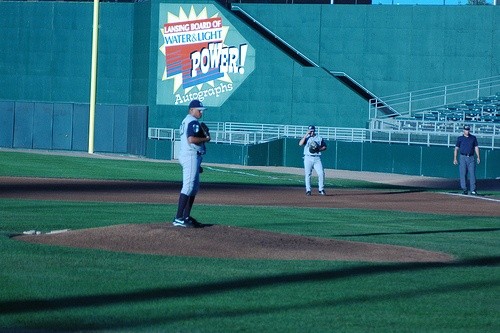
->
[309,141,321,154]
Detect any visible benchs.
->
[395,92,500,134]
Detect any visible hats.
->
[464,125,470,130]
[308,125,315,131]
[189,100,207,111]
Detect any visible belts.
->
[461,153,474,157]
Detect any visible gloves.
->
[206,133,211,141]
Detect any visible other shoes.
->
[319,190,326,195]
[471,191,477,195]
[462,191,468,195]
[185,217,196,225]
[173,217,194,228]
[306,191,311,196]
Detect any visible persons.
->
[172,100,211,228]
[453,125,481,196]
[299,125,327,197]
